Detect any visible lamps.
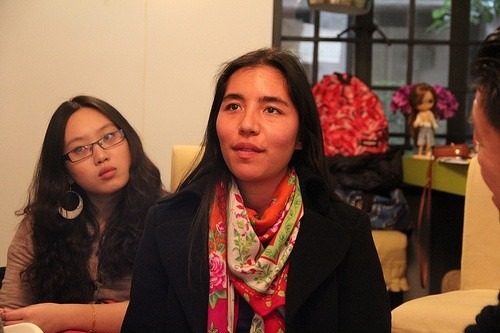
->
[312,73,390,157]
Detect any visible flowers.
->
[390,84,457,120]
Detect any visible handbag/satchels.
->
[432,142,471,160]
[334,184,416,232]
[328,147,403,214]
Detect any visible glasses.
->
[62,128,126,163]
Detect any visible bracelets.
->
[87,303,97,332]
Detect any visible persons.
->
[118,48,394,333]
[464,26,500,333]
[0,96,173,333]
[407,83,439,158]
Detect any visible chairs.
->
[391,154,499,332]
